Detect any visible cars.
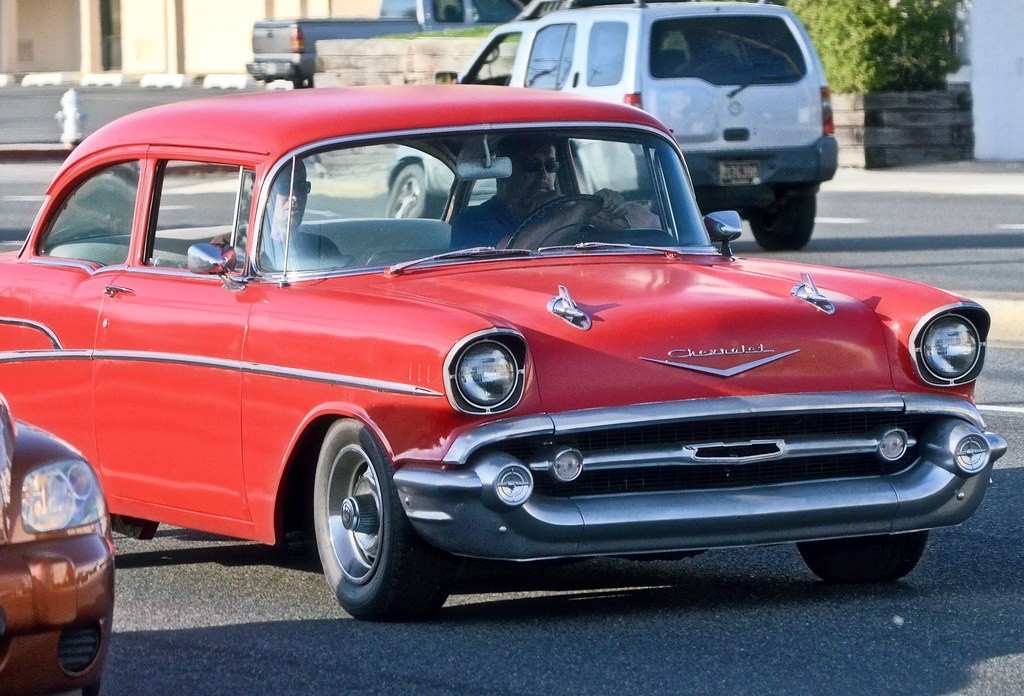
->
[0,81,1007,625]
[0,395,116,696]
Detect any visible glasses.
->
[275,180,312,195]
[521,157,561,174]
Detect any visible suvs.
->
[382,1,840,250]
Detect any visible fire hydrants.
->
[55,87,86,144]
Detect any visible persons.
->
[208,157,345,278]
[449,131,630,250]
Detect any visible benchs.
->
[49,217,449,269]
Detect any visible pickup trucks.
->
[246,0,525,90]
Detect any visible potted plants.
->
[785,0,975,169]
